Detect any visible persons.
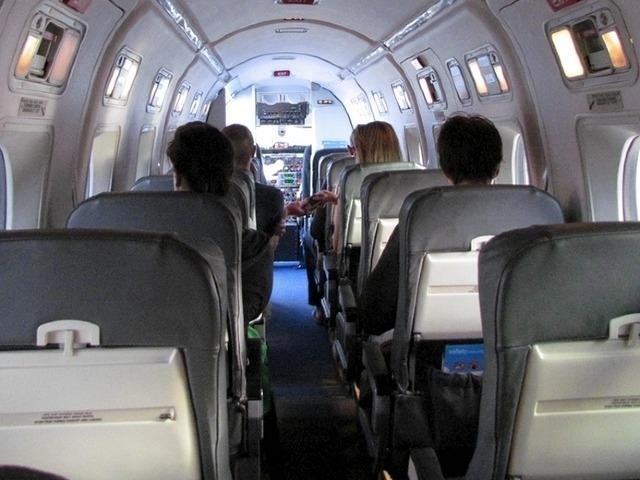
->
[358,116,503,336]
[313,121,404,326]
[167,122,280,463]
[205,124,307,249]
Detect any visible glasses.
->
[347,144,358,155]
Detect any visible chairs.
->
[356,187,567,462]
[392,222,640,480]
[0,230,229,480]
[308,146,453,387]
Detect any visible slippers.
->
[311,309,327,324]
